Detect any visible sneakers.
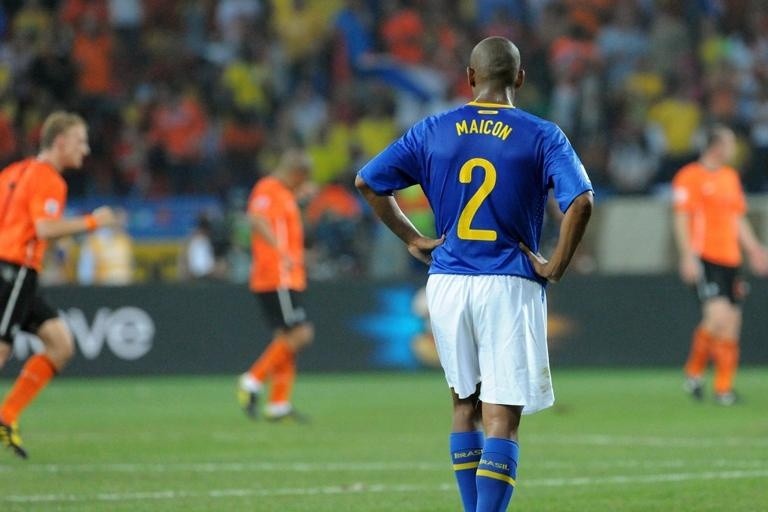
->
[681,376,705,400]
[2,424,30,460]
[234,373,255,418]
[714,393,742,406]
[265,405,305,425]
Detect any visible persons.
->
[354,35,595,511]
[669,121,767,409]
[236,152,314,427]
[0,108,118,461]
[1,2,764,290]
